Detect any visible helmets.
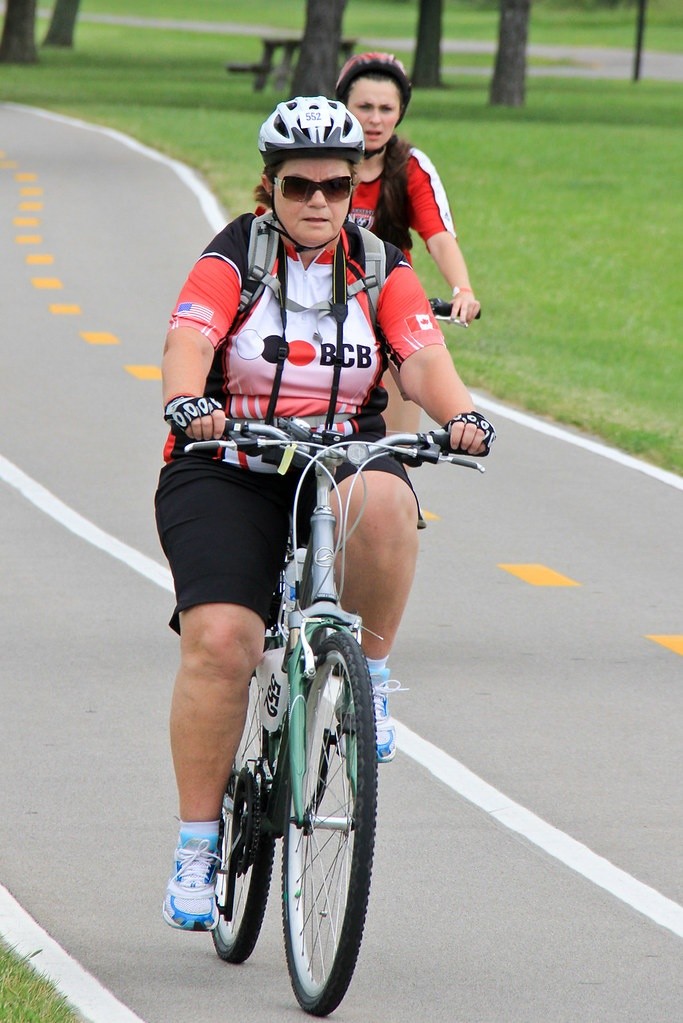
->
[336,51,414,111]
[260,95,364,166]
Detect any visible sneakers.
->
[336,669,395,763]
[165,838,220,932]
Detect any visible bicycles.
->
[179,413,487,1017]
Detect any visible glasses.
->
[272,174,354,203]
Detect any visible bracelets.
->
[450,287,473,296]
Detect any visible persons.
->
[326,53,484,329]
[152,92,496,934]
